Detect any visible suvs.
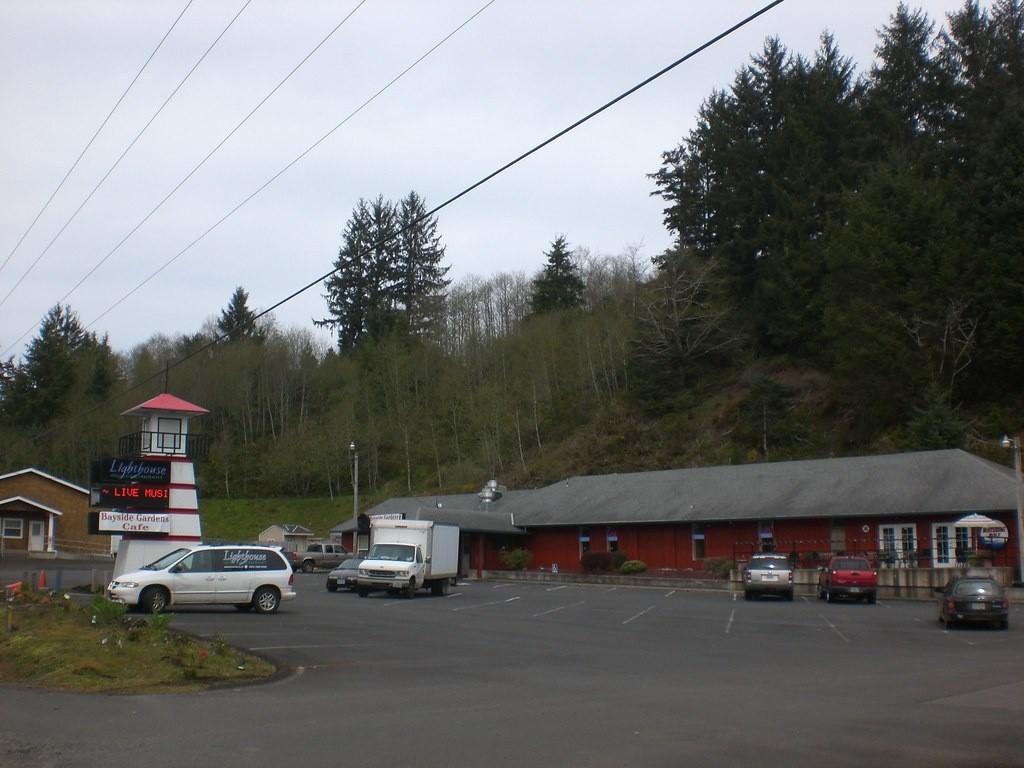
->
[742,552,797,600]
[934,574,1010,629]
[106,542,297,615]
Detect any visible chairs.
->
[788,551,923,569]
[953,547,998,568]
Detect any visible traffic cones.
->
[38,568,48,589]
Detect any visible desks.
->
[965,549,993,566]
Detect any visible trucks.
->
[357,519,460,600]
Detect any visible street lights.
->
[1001,434,1024,583]
[349,440,359,559]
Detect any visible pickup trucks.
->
[286,542,363,573]
[816,555,879,605]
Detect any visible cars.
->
[326,558,365,593]
